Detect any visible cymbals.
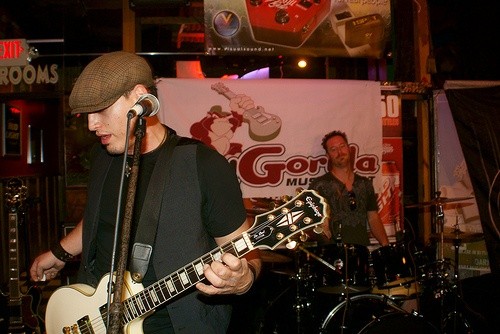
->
[428,232,483,240]
[405,196,475,209]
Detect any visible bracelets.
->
[50,242,75,263]
[247,263,256,280]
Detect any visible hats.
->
[68,51,153,114]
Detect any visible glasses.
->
[348,191,357,210]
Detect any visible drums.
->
[372,240,426,289]
[320,294,422,334]
[428,273,455,301]
[310,244,369,296]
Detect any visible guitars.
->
[44,187,330,334]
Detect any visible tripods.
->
[444,241,476,334]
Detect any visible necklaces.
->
[125,123,166,178]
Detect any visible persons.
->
[309,130,389,246]
[31,52,261,334]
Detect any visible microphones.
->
[394,220,401,242]
[127,94,160,119]
